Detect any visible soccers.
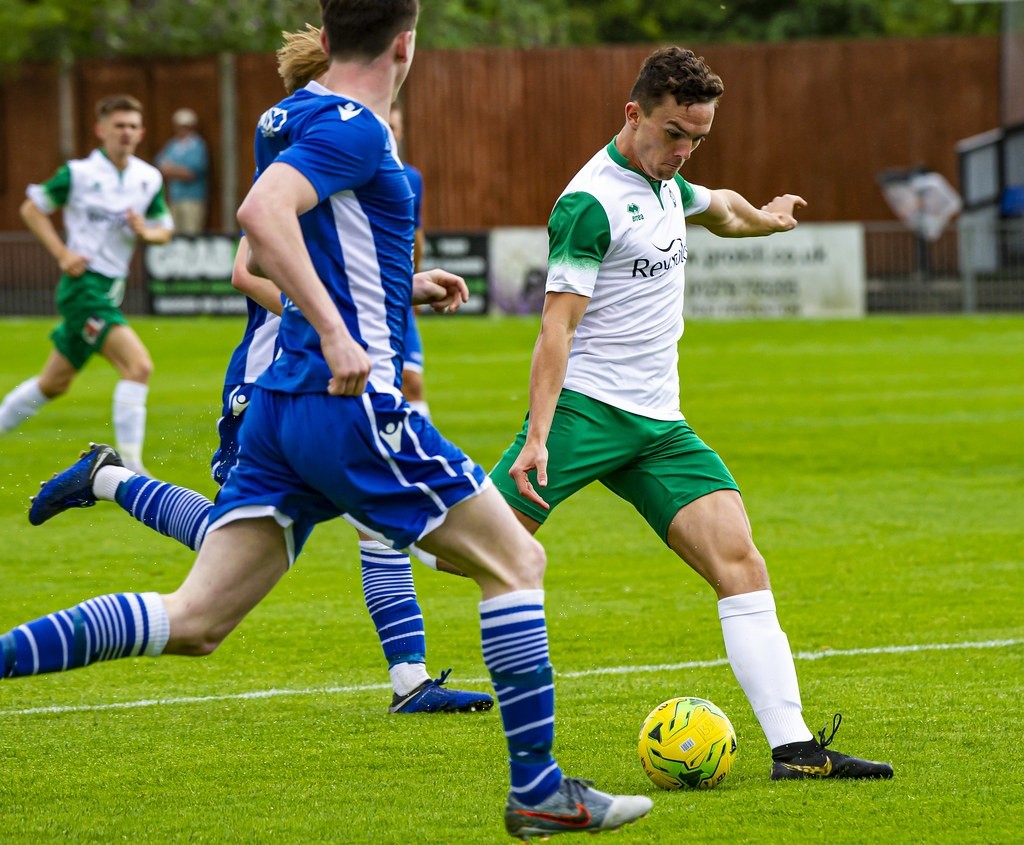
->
[638,697,737,791]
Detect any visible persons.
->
[27,16,494,716]
[154,109,208,237]
[0,0,653,840]
[389,100,423,319]
[1,93,175,485]
[344,47,894,783]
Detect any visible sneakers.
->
[386,679,497,712]
[503,786,654,840]
[29,440,122,528]
[770,746,892,781]
